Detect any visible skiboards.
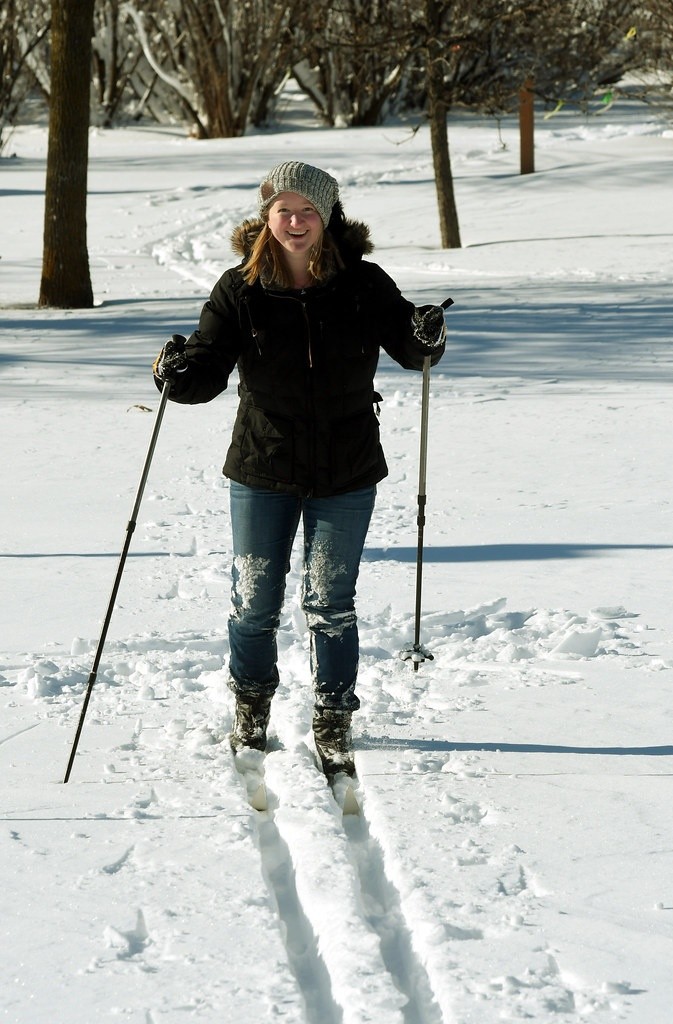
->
[248,785,361,816]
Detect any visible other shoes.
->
[226,680,276,752]
[314,706,357,778]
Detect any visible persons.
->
[150,158,446,779]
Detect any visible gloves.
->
[412,305,446,347]
[154,333,190,378]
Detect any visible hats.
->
[256,162,339,232]
